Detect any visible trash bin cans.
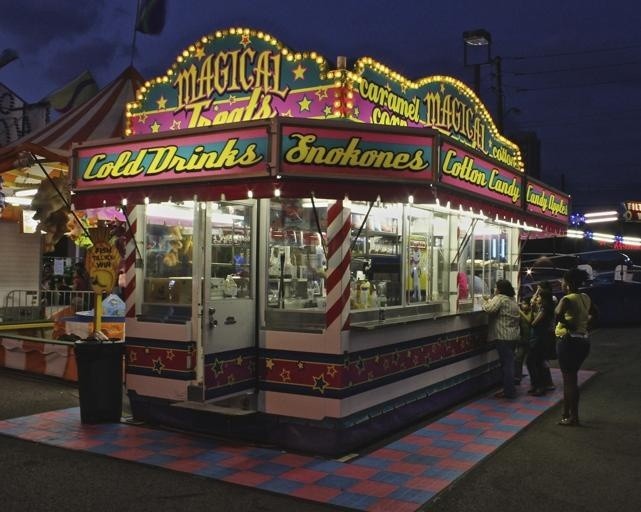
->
[75,338,125,423]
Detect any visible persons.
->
[41,269,85,305]
[554,271,600,425]
[481,280,556,398]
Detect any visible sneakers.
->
[558,413,579,426]
[527,386,554,396]
[495,392,505,398]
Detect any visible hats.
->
[565,269,582,290]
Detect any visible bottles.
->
[350,272,378,310]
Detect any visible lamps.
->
[129,0,167,66]
[462,26,494,70]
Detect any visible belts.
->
[561,333,588,339]
